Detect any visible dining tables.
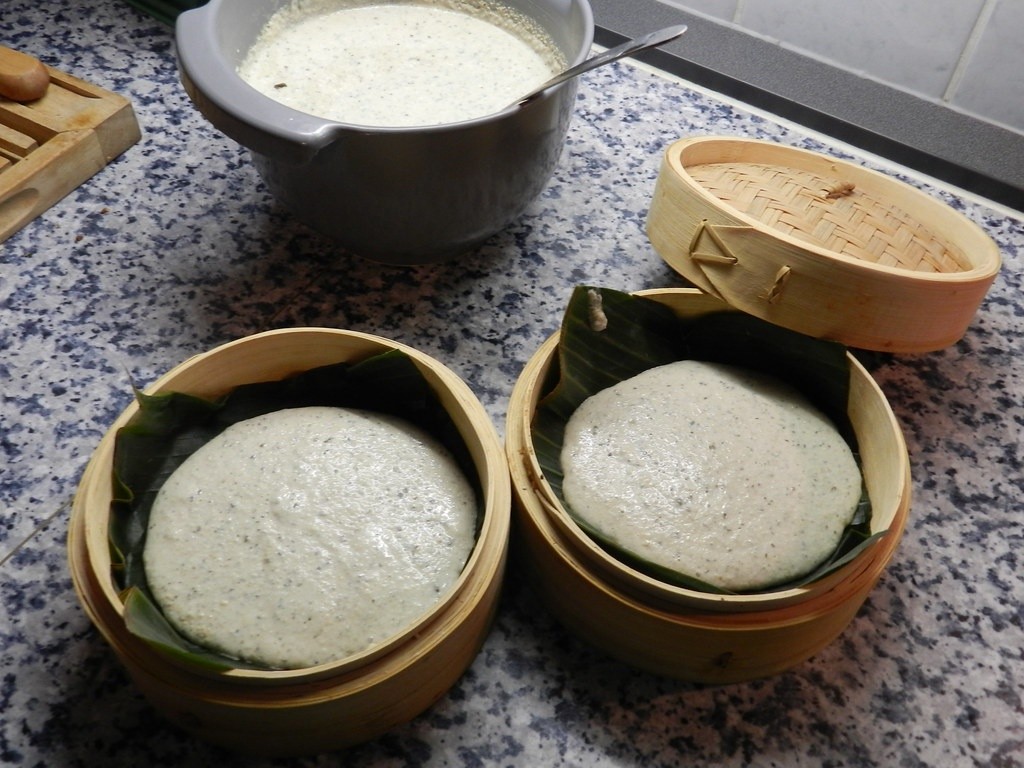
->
[1,2,1024,768]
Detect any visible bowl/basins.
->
[176,0,594,255]
